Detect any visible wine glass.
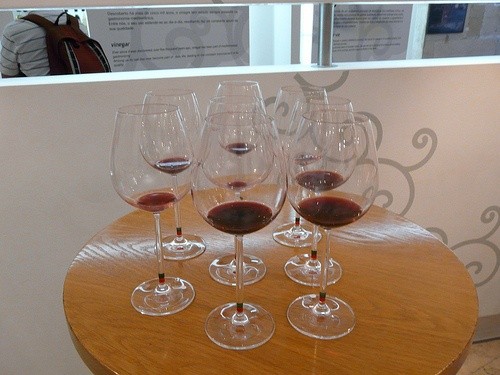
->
[108,80,379,351]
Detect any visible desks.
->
[62,181,480,375]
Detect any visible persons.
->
[1,9,89,77]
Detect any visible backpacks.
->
[20,12,111,76]
